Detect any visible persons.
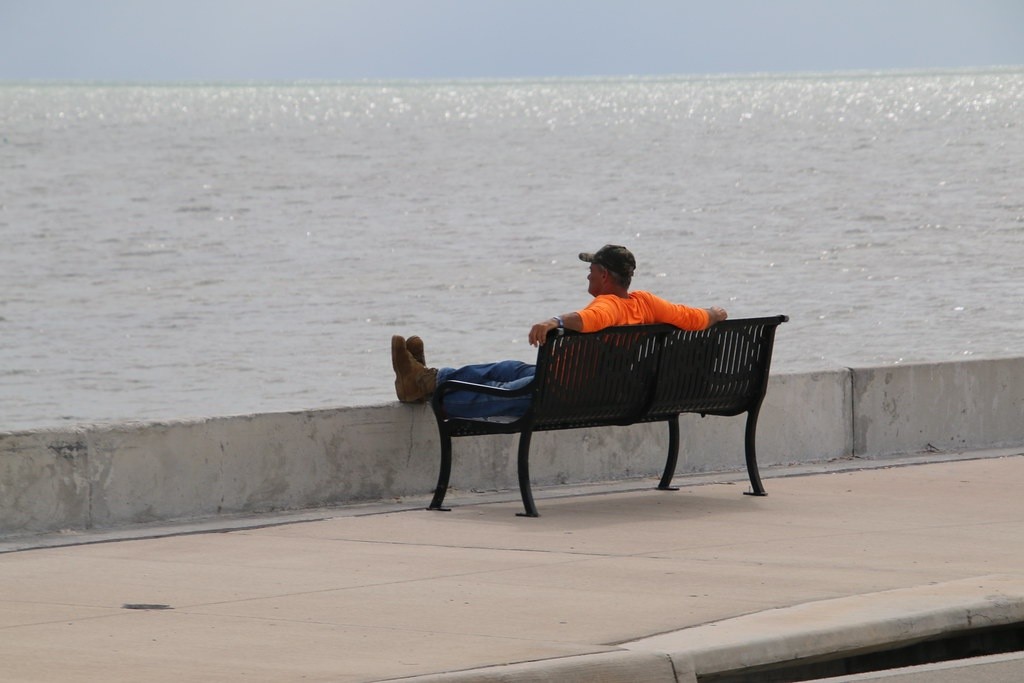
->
[390,244,728,406]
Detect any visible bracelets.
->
[552,316,564,328]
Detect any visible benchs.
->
[427,313,790,518]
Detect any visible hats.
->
[578,245,636,276]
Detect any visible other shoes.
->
[406,335,426,402]
[391,336,437,403]
